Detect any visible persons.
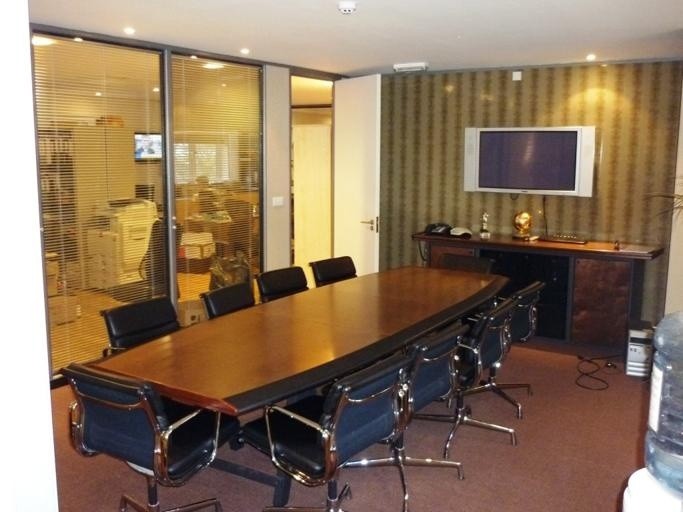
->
[134,136,155,156]
[193,177,224,212]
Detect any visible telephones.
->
[426,223,449,234]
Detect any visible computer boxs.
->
[626,320,654,378]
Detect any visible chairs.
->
[200,281,256,323]
[225,198,260,270]
[57,361,224,512]
[342,324,468,512]
[437,281,544,419]
[240,351,406,512]
[310,256,358,288]
[95,295,184,357]
[256,266,310,305]
[411,297,520,459]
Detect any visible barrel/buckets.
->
[643,311,683,497]
[627,327,651,376]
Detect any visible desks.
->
[412,231,665,363]
[81,267,508,509]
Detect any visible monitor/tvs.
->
[134,132,162,164]
[464,126,596,198]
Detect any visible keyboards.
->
[538,234,587,245]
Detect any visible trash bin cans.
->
[49,291,77,325]
